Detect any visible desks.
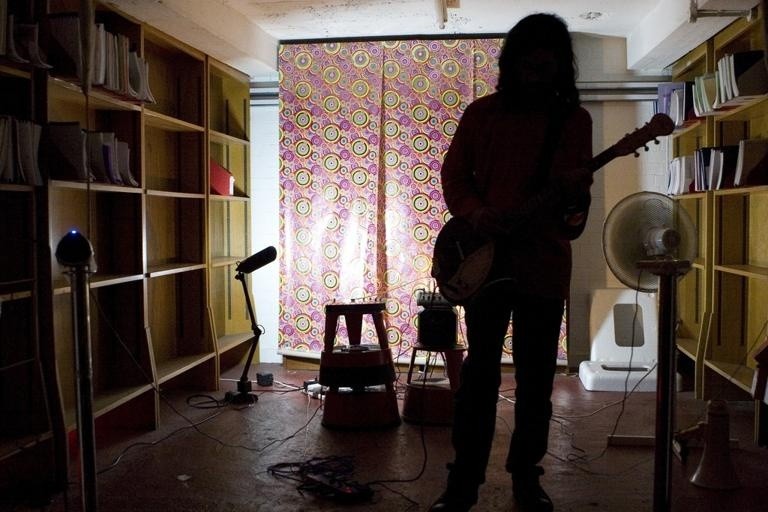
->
[402,345,467,432]
[319,301,402,433]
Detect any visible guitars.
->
[431,114,675,305]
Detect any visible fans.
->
[601,193,698,512]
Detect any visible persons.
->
[428,12,595,512]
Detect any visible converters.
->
[302,379,316,389]
[307,383,322,392]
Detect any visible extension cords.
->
[300,386,326,401]
[301,463,375,503]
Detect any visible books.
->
[1,0,158,104]
[665,137,768,196]
[0,113,141,187]
[653,48,768,134]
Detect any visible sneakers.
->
[514,484,553,511]
[430,487,478,511]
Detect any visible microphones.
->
[235,246,276,272]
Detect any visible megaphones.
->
[688,398,742,490]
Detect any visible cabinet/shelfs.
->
[0,0,260,512]
[667,7,768,446]
